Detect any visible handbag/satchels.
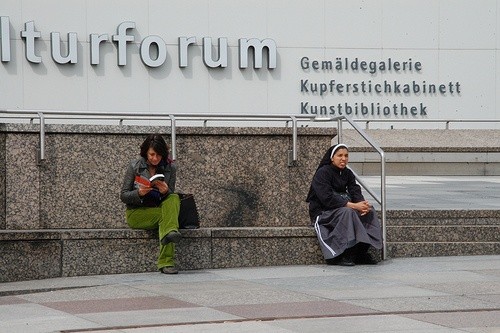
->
[178,192,201,229]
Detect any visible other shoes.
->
[355,253,378,264]
[161,267,178,274]
[326,254,355,266]
[161,230,182,245]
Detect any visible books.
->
[134,174,165,188]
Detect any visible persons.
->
[120,133,182,274]
[305,144,383,265]
